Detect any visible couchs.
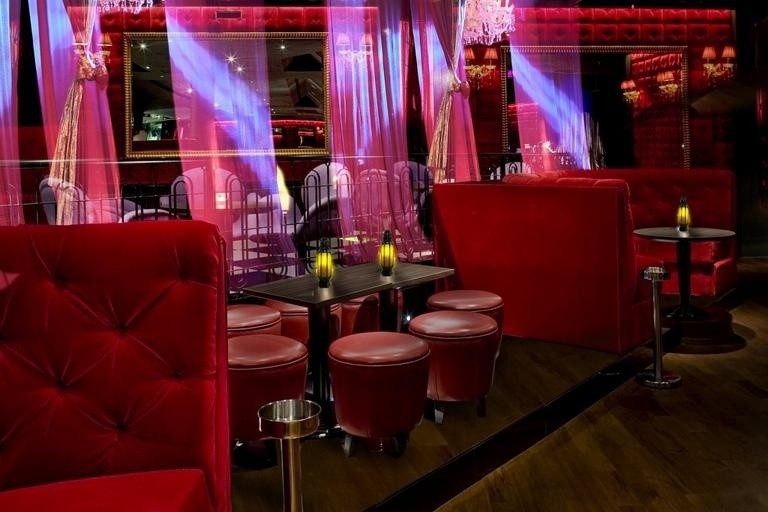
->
[433,166,736,355]
[269,120,325,151]
[0,221,235,512]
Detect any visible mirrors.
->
[499,45,690,171]
[122,31,332,158]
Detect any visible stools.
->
[227,334,309,457]
[409,310,500,418]
[227,303,281,341]
[265,299,342,375]
[428,289,505,349]
[342,291,404,339]
[328,331,433,458]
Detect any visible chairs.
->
[389,162,434,194]
[160,167,245,211]
[491,162,532,182]
[117,208,183,221]
[354,171,412,241]
[95,198,140,222]
[40,179,111,223]
[302,163,353,209]
[252,195,350,275]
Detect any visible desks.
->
[634,226,737,347]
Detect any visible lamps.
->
[376,230,396,275]
[676,197,691,232]
[656,72,678,96]
[620,80,639,104]
[72,32,113,79]
[462,47,498,91]
[702,46,736,85]
[312,238,336,284]
[335,32,374,76]
